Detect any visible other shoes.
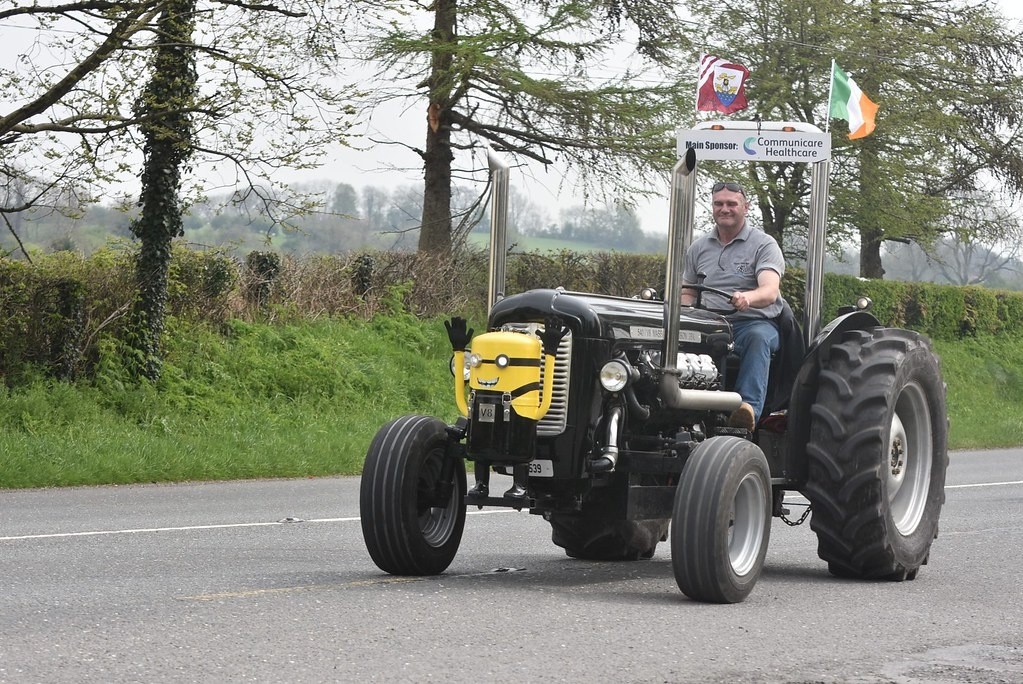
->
[728,402,755,433]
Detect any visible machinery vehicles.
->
[358,118,949,605]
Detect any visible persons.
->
[680,183,786,434]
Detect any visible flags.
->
[697,54,749,114]
[828,63,880,140]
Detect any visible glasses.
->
[712,182,746,198]
